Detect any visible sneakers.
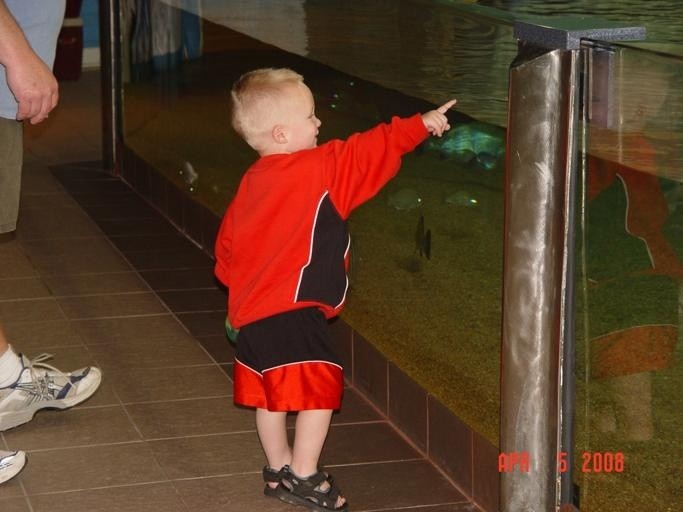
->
[0,350,103,433]
[0,446,28,485]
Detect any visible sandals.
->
[261,463,286,499]
[273,464,350,512]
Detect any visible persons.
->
[0,1,103,488]
[208,65,458,510]
[576,54,681,457]
